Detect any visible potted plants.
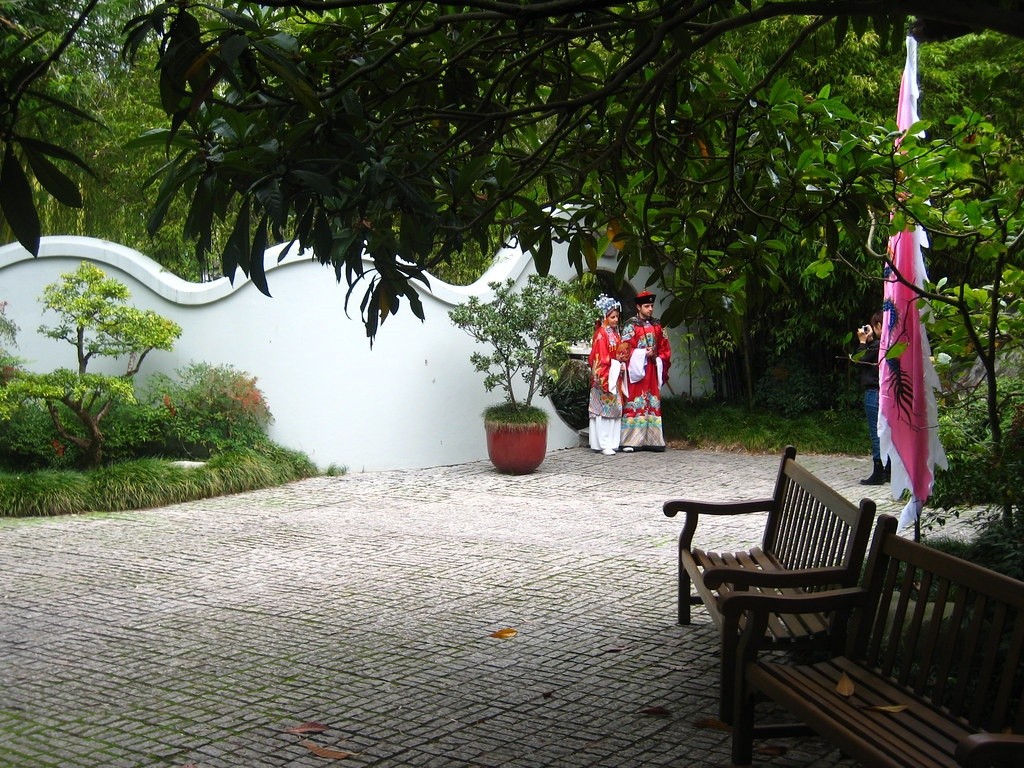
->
[447,274,601,475]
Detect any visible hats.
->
[593,293,622,351]
[634,283,656,304]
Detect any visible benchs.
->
[715,513,1024,768]
[663,446,877,725]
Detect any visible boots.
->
[884,456,891,483]
[860,458,887,485]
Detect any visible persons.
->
[621,286,672,452]
[853,310,891,485]
[588,294,629,456]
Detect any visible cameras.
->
[862,326,869,333]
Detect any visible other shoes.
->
[651,445,664,452]
[623,447,633,452]
[602,449,616,455]
